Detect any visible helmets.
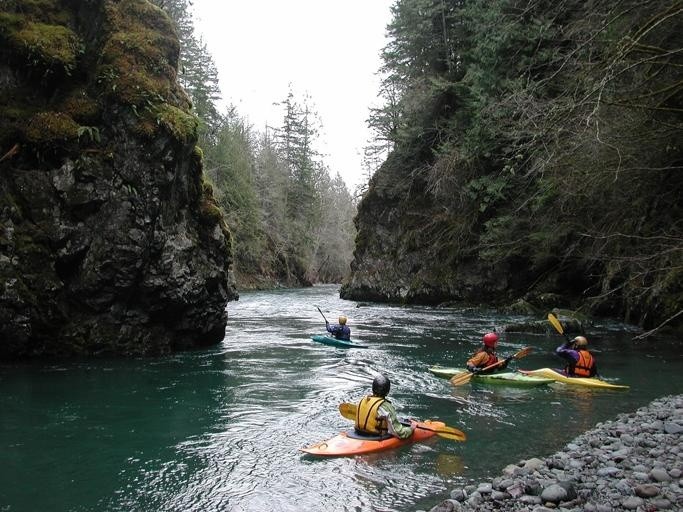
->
[572,336,588,347]
[372,376,390,396]
[339,316,347,324]
[484,333,498,348]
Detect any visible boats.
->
[311,334,369,351]
[516,367,630,389]
[429,365,556,387]
[297,418,446,458]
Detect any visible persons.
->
[354,375,419,441]
[465,332,512,375]
[554,334,598,378]
[324,316,350,341]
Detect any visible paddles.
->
[547,312,601,376]
[339,401,466,441]
[450,348,528,386]
